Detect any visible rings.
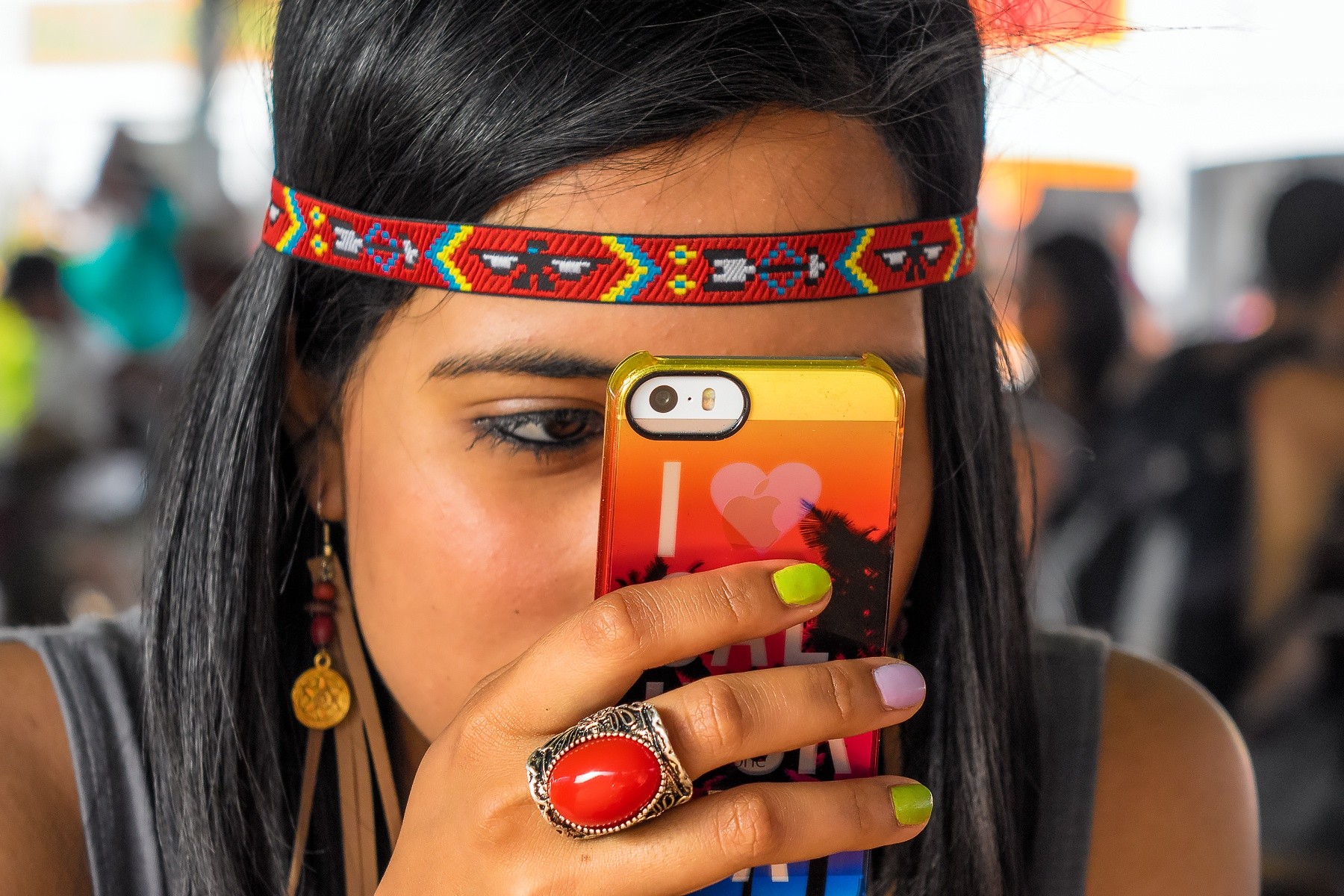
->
[523,702,693,835]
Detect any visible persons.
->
[1,161,240,625]
[1001,173,1344,896]
[2,0,1263,895]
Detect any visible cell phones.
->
[586,348,905,896]
